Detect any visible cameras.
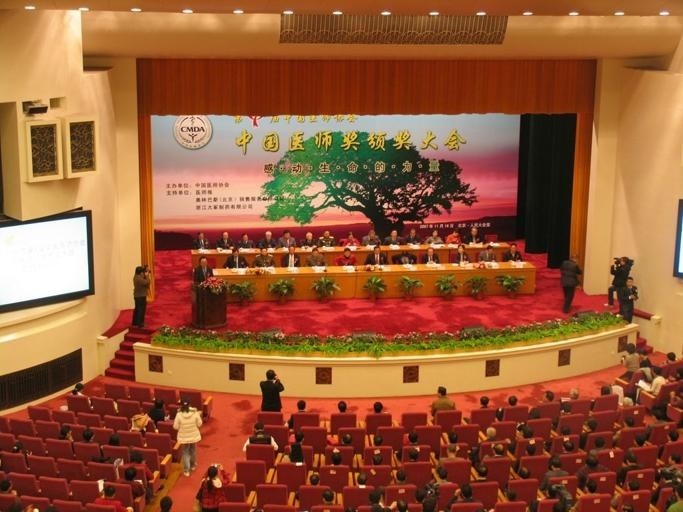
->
[143,264,151,274]
[633,290,639,300]
[614,257,634,267]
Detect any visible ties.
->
[289,254,295,267]
[375,254,380,264]
[234,256,238,268]
[428,255,433,261]
[224,239,227,248]
[202,265,206,281]
[200,239,204,248]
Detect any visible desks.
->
[356,261,536,298]
[190,243,449,268]
[212,265,355,304]
[450,241,510,261]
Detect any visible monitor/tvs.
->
[674,198,683,279]
[0,210,96,312]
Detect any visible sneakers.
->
[136,323,148,328]
[616,314,625,319]
[603,304,614,308]
[183,472,190,476]
[191,461,198,471]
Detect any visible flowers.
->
[199,275,228,296]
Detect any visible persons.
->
[485,444,505,460]
[281,246,301,267]
[446,431,458,444]
[241,422,278,453]
[512,444,537,474]
[645,408,666,442]
[191,232,210,250]
[123,450,155,482]
[611,479,640,509]
[318,230,336,247]
[259,230,277,248]
[287,399,307,429]
[622,396,634,408]
[46,502,58,512]
[604,256,635,318]
[475,464,489,483]
[445,227,462,244]
[415,488,434,512]
[367,487,398,512]
[506,492,518,502]
[577,418,599,450]
[495,407,506,422]
[502,243,523,262]
[655,453,681,480]
[130,408,159,447]
[422,247,439,264]
[464,226,486,244]
[308,474,320,486]
[81,429,101,442]
[551,401,572,431]
[403,227,422,244]
[356,472,370,487]
[651,469,680,506]
[527,407,541,421]
[396,431,420,461]
[61,425,76,454]
[100,433,122,452]
[158,496,175,511]
[118,467,153,504]
[147,398,170,429]
[371,435,383,446]
[482,426,498,443]
[215,231,235,249]
[570,479,602,512]
[477,395,490,410]
[396,470,407,484]
[635,367,666,403]
[95,485,135,512]
[342,232,360,246]
[10,441,32,468]
[259,370,285,412]
[443,443,461,462]
[361,230,380,246]
[193,255,213,284]
[611,416,635,447]
[445,483,475,511]
[589,437,608,457]
[340,432,353,447]
[0,479,18,494]
[396,499,408,512]
[657,430,680,458]
[528,484,572,512]
[589,384,613,411]
[133,266,152,327]
[71,382,92,408]
[334,248,358,266]
[373,401,383,413]
[455,244,471,262]
[539,457,569,491]
[661,351,677,364]
[517,465,530,481]
[319,491,335,506]
[670,387,683,410]
[540,391,555,402]
[620,277,639,323]
[328,452,342,474]
[545,426,573,451]
[223,248,249,268]
[425,229,444,243]
[507,423,536,455]
[621,503,634,512]
[633,433,650,449]
[196,463,230,512]
[559,254,582,313]
[338,401,347,414]
[563,442,576,454]
[578,454,609,489]
[479,245,496,261]
[344,507,357,512]
[277,230,298,248]
[251,248,275,268]
[300,232,318,248]
[668,367,683,381]
[238,232,254,248]
[618,343,640,381]
[407,446,420,464]
[370,454,383,465]
[476,508,488,511]
[383,229,402,244]
[639,348,654,368]
[364,247,389,265]
[561,387,579,405]
[666,483,682,512]
[304,248,326,266]
[173,395,203,478]
[616,452,640,484]
[288,431,306,463]
[431,385,456,420]
[506,394,519,407]
[6,499,33,512]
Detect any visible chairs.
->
[508,391,682,511]
[206,410,507,511]
[615,351,683,420]
[0,381,213,506]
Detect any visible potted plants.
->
[433,271,462,300]
[461,274,488,300]
[361,272,386,301]
[395,273,418,298]
[494,272,525,299]
[307,273,342,304]
[225,278,258,309]
[265,277,295,305]
